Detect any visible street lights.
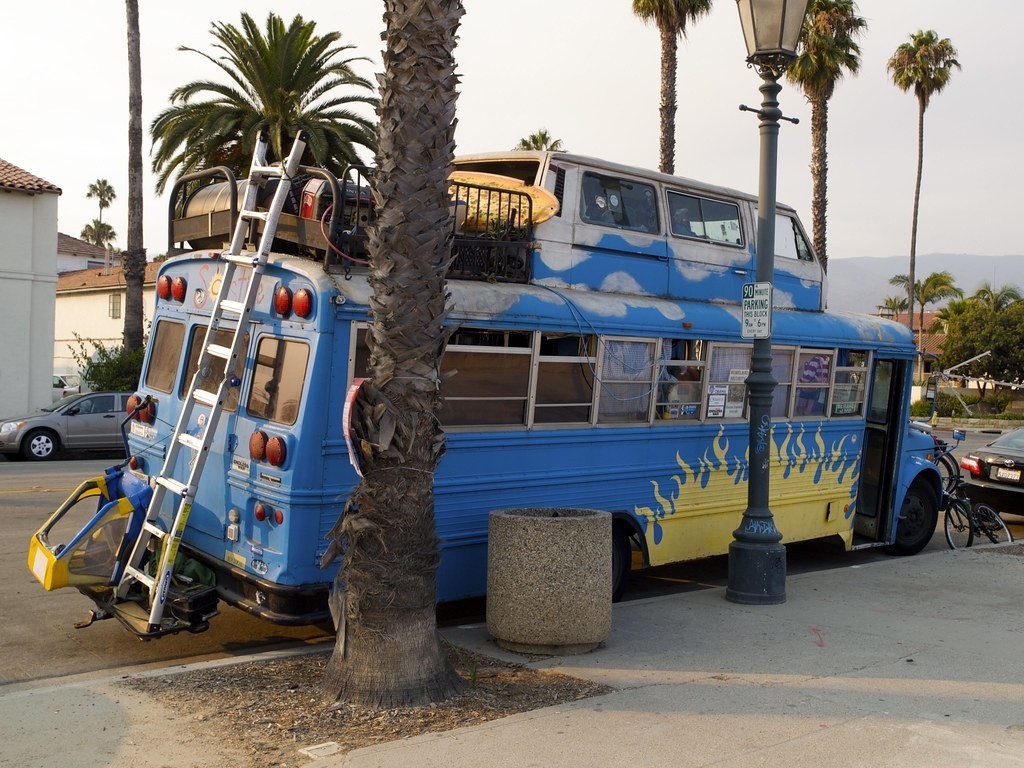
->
[725,1,812,607]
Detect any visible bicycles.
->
[929,432,960,495]
[940,475,1015,549]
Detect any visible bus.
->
[27,150,968,642]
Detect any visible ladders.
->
[114,121,310,638]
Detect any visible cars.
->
[956,428,1024,522]
[0,390,133,462]
